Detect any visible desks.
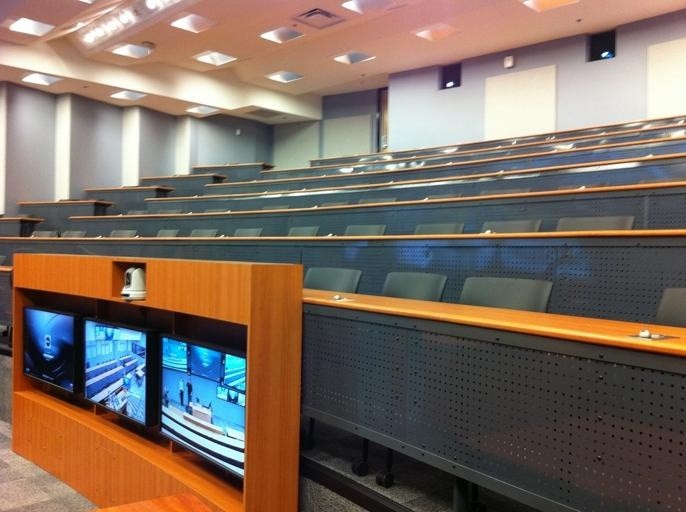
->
[0,112,684,510]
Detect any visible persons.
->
[186,378,193,407]
[178,377,184,407]
[164,389,169,408]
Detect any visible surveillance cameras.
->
[121,267,146,300]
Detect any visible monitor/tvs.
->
[157,332,247,482]
[22,306,81,394]
[81,316,157,428]
